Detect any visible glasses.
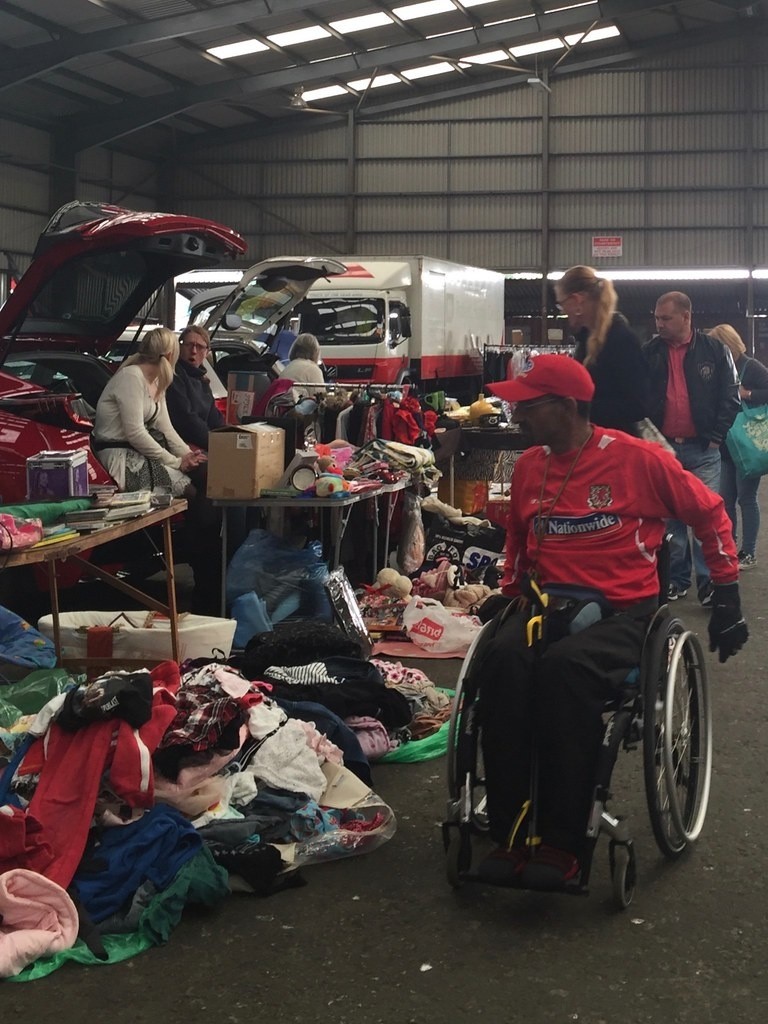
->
[514,399,557,414]
[555,290,580,311]
[181,342,208,351]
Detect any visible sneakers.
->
[666,584,689,601]
[697,587,715,606]
[737,550,759,571]
[476,845,582,883]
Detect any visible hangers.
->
[484,343,578,356]
[288,382,412,412]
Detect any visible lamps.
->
[525,54,554,97]
[289,83,309,129]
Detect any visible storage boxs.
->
[25,450,89,502]
[205,421,287,502]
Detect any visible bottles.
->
[471,395,494,419]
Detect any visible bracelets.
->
[748,390,752,401]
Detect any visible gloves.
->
[707,585,750,664]
[475,594,510,624]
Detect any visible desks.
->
[211,428,527,618]
[0,499,189,670]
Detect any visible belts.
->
[665,437,698,444]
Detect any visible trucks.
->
[292,255,506,408]
[104,322,182,379]
[182,286,298,387]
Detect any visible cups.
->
[512,329,524,344]
[290,465,319,492]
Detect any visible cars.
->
[114,255,348,427]
[1,200,249,595]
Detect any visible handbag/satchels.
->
[150,428,170,452]
[418,508,509,581]
[437,481,489,515]
[724,405,768,482]
[402,595,484,653]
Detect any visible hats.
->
[485,355,595,402]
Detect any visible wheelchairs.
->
[441,526,713,911]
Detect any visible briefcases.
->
[26,450,89,501]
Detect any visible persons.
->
[641,292,740,605]
[554,266,653,440]
[707,325,768,570]
[93,325,245,570]
[165,326,261,532]
[279,333,326,402]
[476,354,748,890]
